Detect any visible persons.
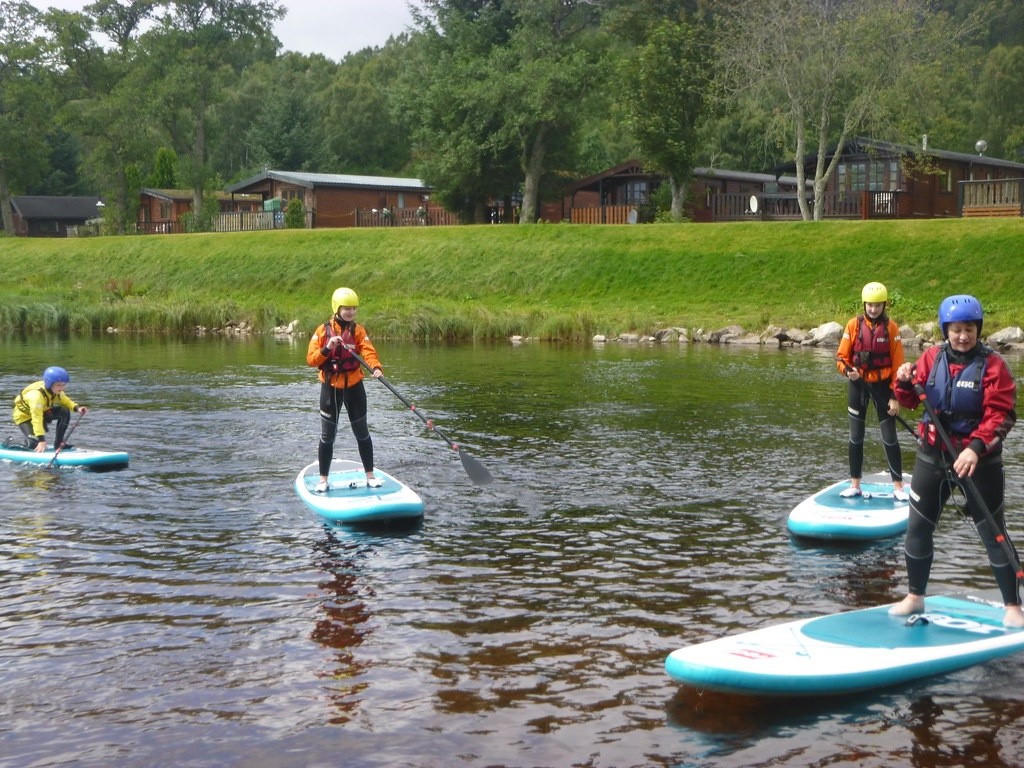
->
[306,286,382,492]
[2,366,89,453]
[887,295,1024,628]
[837,282,911,501]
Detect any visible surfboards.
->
[0,445,130,466]
[786,472,915,539]
[293,457,424,524]
[664,597,1023,693]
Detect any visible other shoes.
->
[840,487,862,498]
[316,482,329,493]
[2,436,13,448]
[894,488,909,501]
[367,477,382,487]
[55,443,74,450]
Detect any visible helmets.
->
[861,282,888,312]
[331,287,359,314]
[44,366,69,389]
[939,295,983,339]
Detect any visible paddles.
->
[337,338,495,486]
[46,408,86,469]
[910,376,1024,610]
[839,357,920,440]
